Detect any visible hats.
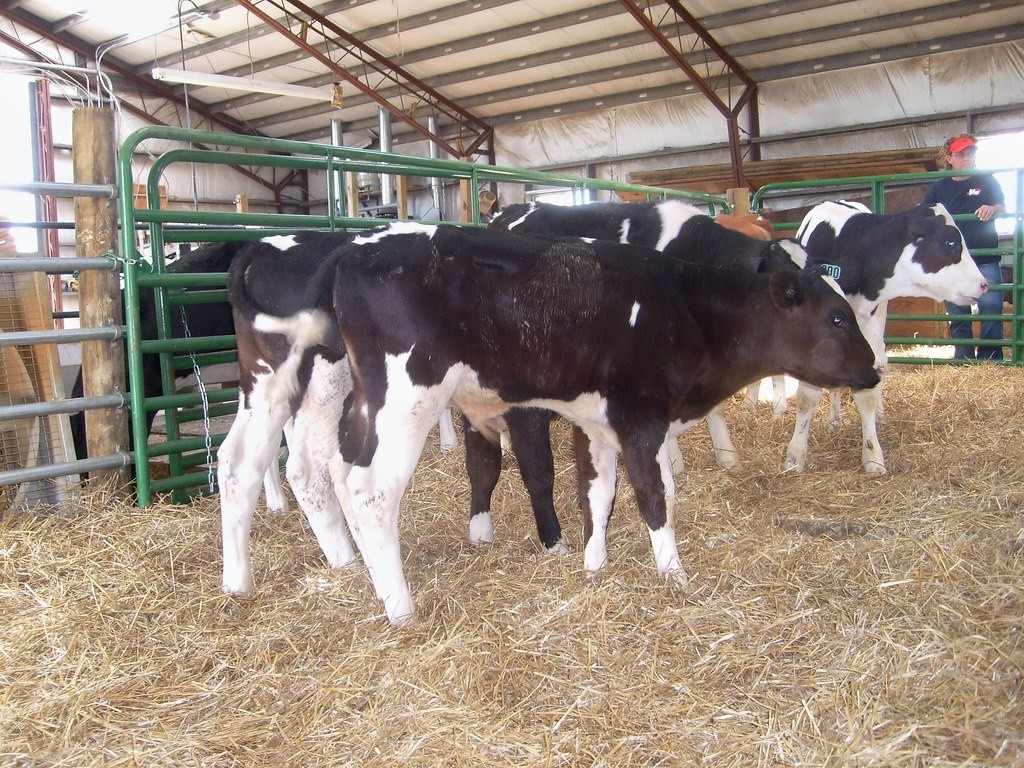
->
[947,137,978,155]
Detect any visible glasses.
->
[950,152,977,157]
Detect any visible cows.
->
[216,216,891,630]
[774,198,988,477]
[70,227,265,501]
[465,199,837,556]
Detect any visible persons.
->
[0,216,16,251]
[923,133,1006,360]
